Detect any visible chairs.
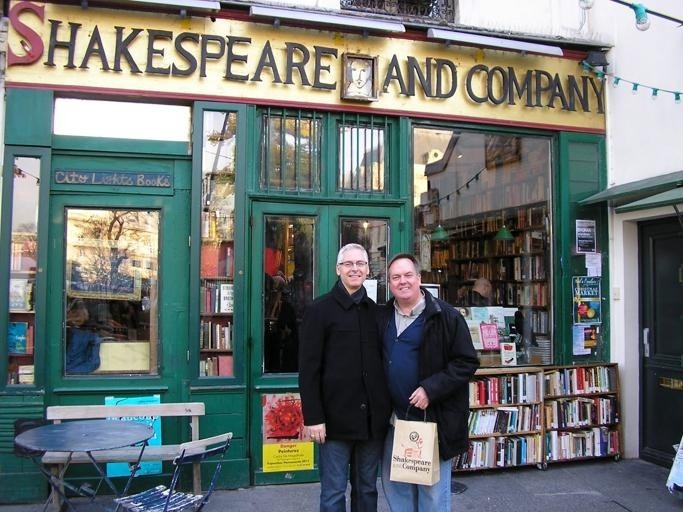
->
[108,429,235,511]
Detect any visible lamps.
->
[491,175,516,242]
[79,0,222,23]
[427,200,450,244]
[245,0,406,40]
[628,1,653,33]
[577,50,610,69]
[420,27,566,61]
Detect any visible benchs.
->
[37,401,207,511]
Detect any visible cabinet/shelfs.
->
[442,364,544,475]
[401,198,552,370]
[6,226,38,369]
[531,361,623,470]
[194,234,236,381]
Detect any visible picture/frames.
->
[61,233,146,305]
[337,49,380,103]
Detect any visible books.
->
[6,233,38,385]
[200,210,234,377]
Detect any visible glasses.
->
[340,261,368,267]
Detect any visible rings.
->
[310,435,314,438]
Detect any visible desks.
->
[12,419,158,512]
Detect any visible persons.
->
[264,275,298,374]
[66,300,101,373]
[345,59,372,97]
[297,244,380,512]
[381,253,480,512]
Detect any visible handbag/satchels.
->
[389,405,440,486]
